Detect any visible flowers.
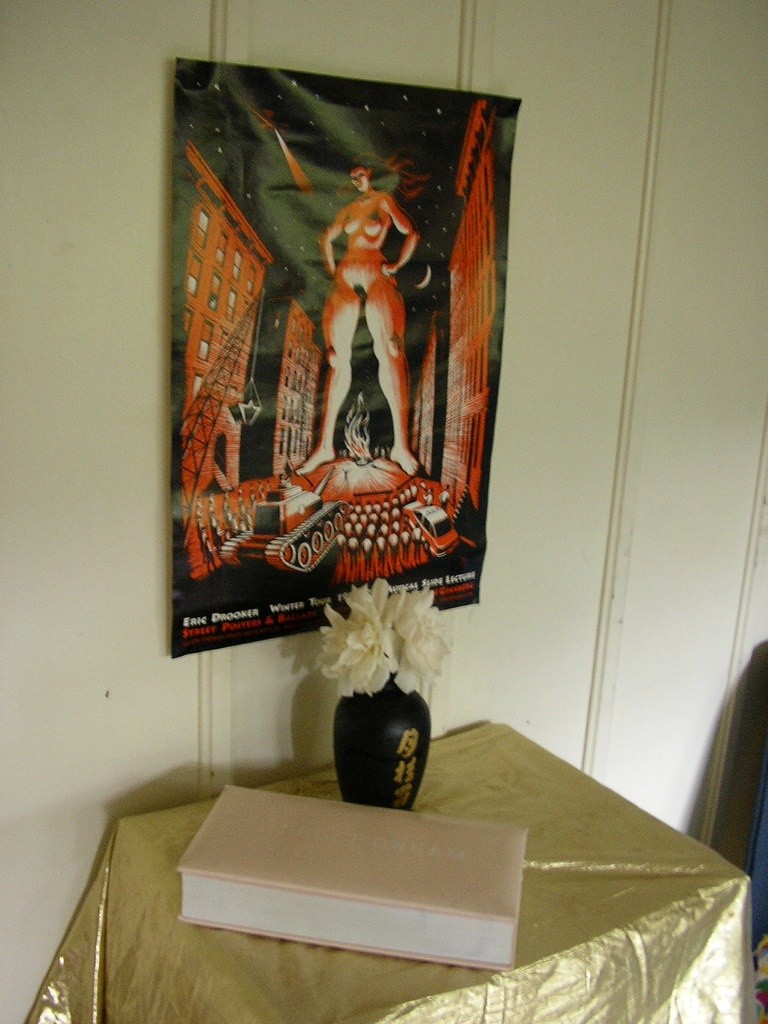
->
[316,578,457,697]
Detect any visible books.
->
[176,783,528,972]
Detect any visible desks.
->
[25,720,758,1024]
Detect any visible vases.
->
[332,670,432,810]
